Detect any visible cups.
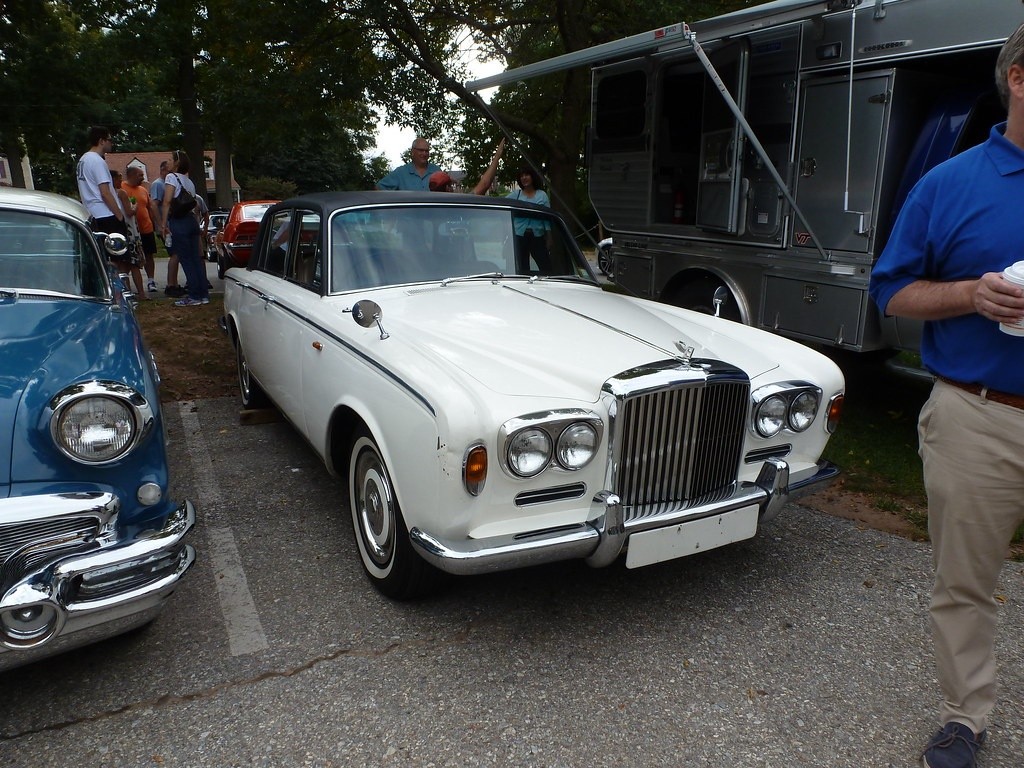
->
[999,260,1024,337]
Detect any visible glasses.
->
[175,149,180,164]
[413,147,429,153]
[102,137,113,142]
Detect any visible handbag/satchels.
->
[171,188,196,218]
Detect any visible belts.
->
[935,375,1024,409]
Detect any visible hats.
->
[429,172,457,190]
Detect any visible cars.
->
[1,184,195,676]
[220,190,850,596]
[216,199,322,280]
[198,212,231,262]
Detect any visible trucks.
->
[464,0,1023,386]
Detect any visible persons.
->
[428,138,507,264]
[505,164,551,271]
[868,23,1024,768]
[77,127,152,302]
[374,137,450,251]
[107,149,213,306]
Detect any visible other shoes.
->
[920,721,988,768]
[164,285,185,296]
[175,297,201,306]
[201,296,210,304]
[139,296,152,302]
[207,279,213,289]
[147,280,158,291]
[184,281,189,293]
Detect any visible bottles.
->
[165,233,173,247]
[131,193,136,204]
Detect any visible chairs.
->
[299,218,379,287]
